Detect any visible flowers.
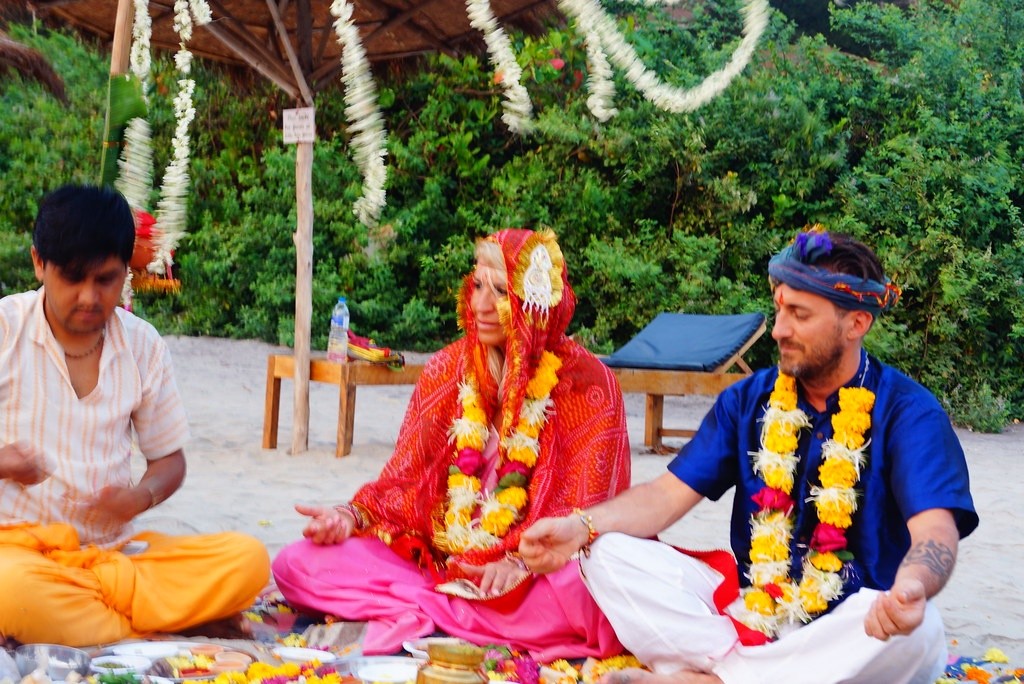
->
[329,0,389,229]
[480,646,646,684]
[112,0,214,309]
[557,0,770,122]
[937,647,1024,684]
[65,605,357,684]
[465,0,533,136]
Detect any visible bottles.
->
[416,643,489,684]
[328,298,350,360]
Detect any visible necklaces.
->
[745,352,876,625]
[63,324,105,359]
[442,342,560,552]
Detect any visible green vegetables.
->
[98,670,141,684]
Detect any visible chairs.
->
[263,312,766,459]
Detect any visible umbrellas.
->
[0,0,560,458]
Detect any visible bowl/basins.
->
[14,642,90,680]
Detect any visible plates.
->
[90,637,574,684]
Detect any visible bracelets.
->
[334,504,364,526]
[574,506,599,544]
[506,550,530,573]
[145,488,157,510]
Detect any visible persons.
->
[522,226,978,684]
[0,183,270,649]
[274,228,658,667]
